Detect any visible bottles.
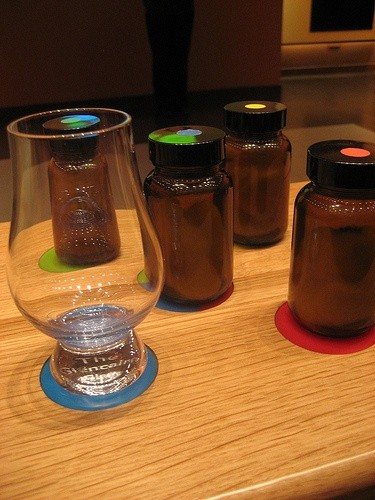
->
[40,115,121,264]
[224,100,292,248]
[138,125,234,303]
[287,137,375,338]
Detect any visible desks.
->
[0,178,375,500]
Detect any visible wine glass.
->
[4,106,166,397]
